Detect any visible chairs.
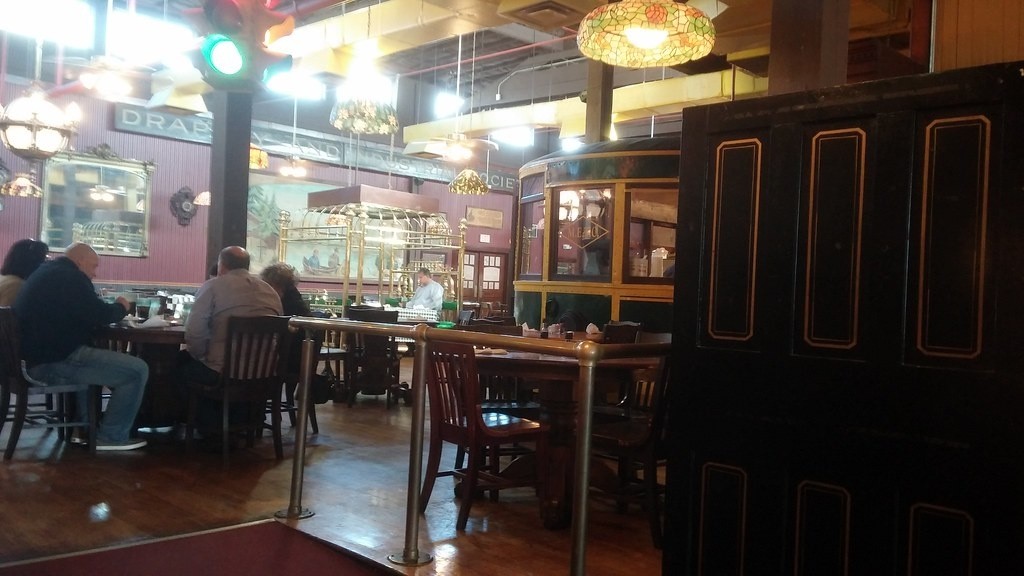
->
[183,314,296,464]
[256,310,331,434]
[0,304,103,458]
[318,296,666,551]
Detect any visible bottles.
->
[541,322,548,339]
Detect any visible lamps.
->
[0,39,73,183]
[1,173,42,198]
[248,142,271,169]
[446,164,489,196]
[329,100,400,134]
[577,0,715,68]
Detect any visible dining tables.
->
[93,316,188,433]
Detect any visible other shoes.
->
[70,427,90,444]
[404,350,414,357]
[95,436,148,452]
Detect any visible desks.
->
[412,348,657,532]
[351,301,435,344]
[310,303,342,315]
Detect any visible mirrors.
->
[40,141,156,258]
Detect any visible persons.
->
[262,266,319,372]
[172,245,283,456]
[209,263,217,280]
[0,238,49,308]
[398,268,444,357]
[12,241,148,451]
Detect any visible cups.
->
[136,298,151,320]
[129,292,145,317]
[629,258,648,277]
[182,303,193,325]
[149,298,160,317]
